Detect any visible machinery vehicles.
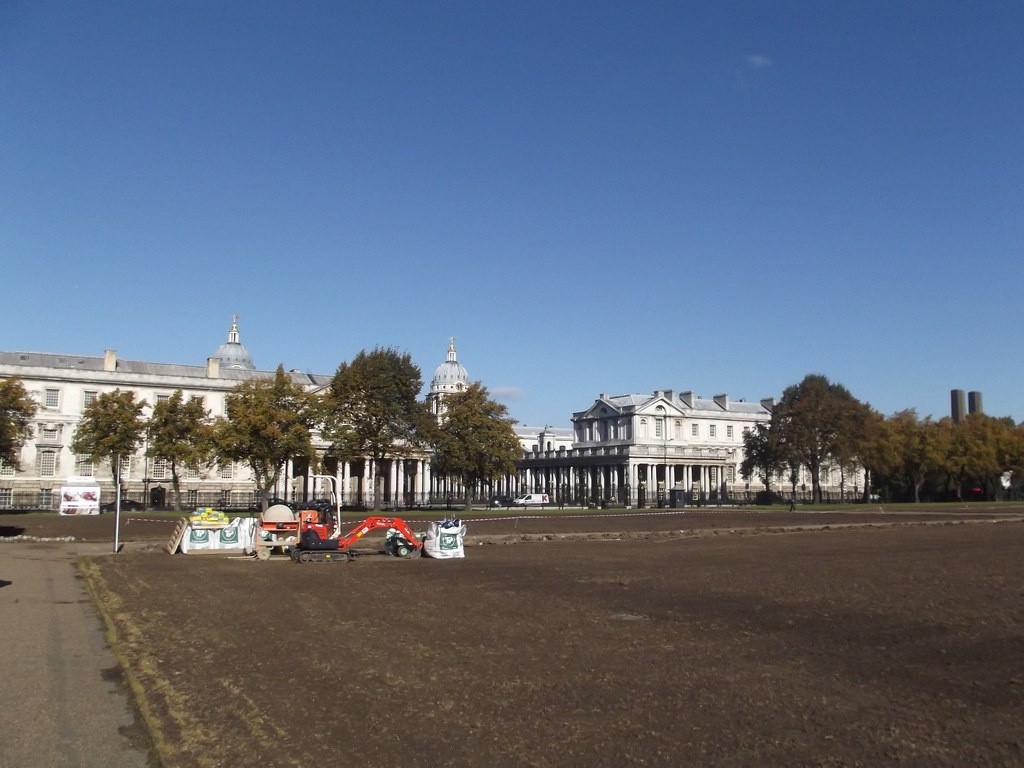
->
[249,473,424,564]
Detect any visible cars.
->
[490,496,506,507]
[100,499,144,514]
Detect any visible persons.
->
[789,494,797,512]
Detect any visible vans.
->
[513,493,550,508]
